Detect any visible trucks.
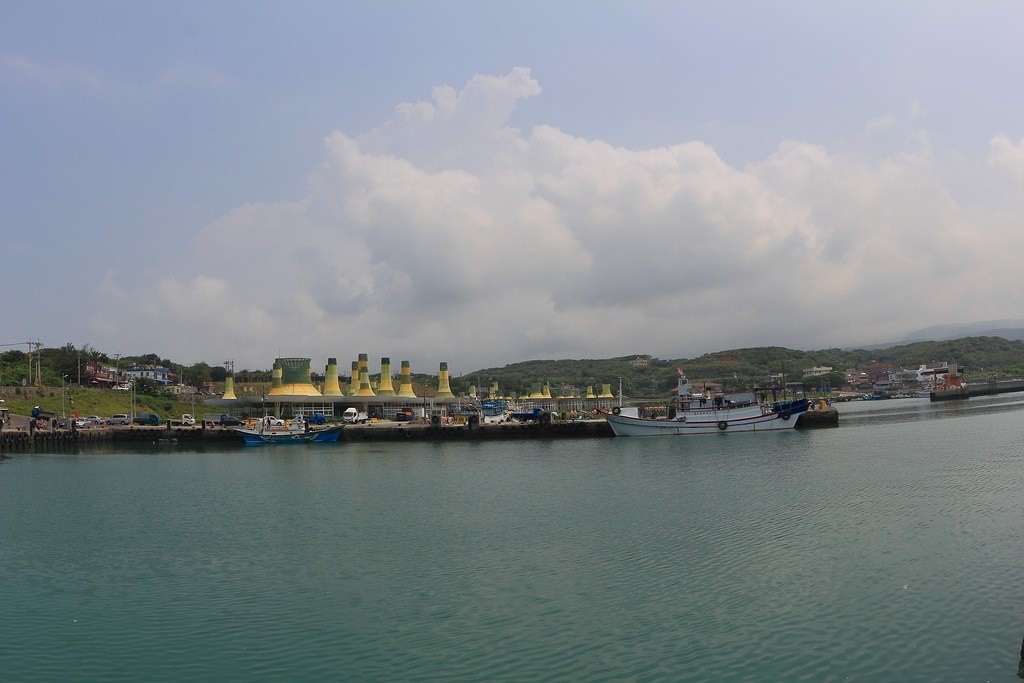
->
[166,414,196,425]
[342,407,369,424]
[30,406,56,419]
[111,384,129,391]
[206,413,230,425]
[296,412,328,425]
[395,407,415,421]
[132,414,163,426]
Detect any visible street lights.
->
[61,372,68,419]
[423,384,429,421]
[124,380,133,423]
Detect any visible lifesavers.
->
[72,420,76,427]
[53,419,58,427]
[612,406,621,414]
[432,415,439,423]
[406,422,587,439]
[2,428,239,446]
[32,418,36,427]
[717,420,727,429]
[471,415,478,423]
[782,409,791,420]
[543,412,550,420]
[292,435,301,439]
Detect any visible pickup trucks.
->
[250,415,285,426]
[510,407,560,422]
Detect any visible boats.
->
[591,366,812,438]
[227,386,347,443]
[823,388,919,404]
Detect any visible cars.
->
[75,418,92,429]
[86,415,105,424]
[193,391,225,396]
[57,419,69,426]
[105,414,131,425]
[219,416,245,427]
